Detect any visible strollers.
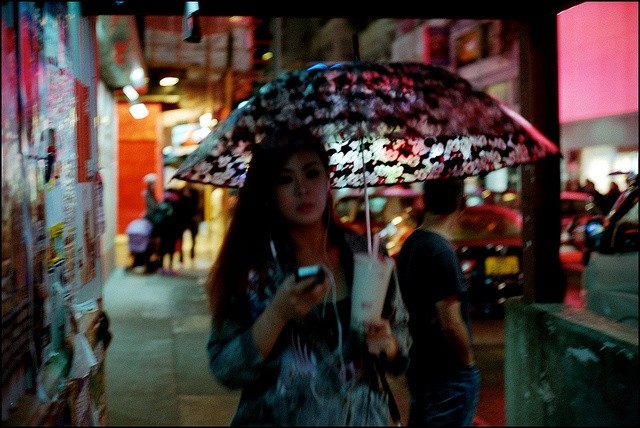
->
[126,219,153,274]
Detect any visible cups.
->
[348,252,396,341]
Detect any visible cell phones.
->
[294,264,324,295]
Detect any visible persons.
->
[128,174,204,274]
[397,178,482,427]
[203,127,413,428]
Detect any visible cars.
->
[333,197,382,253]
[376,204,567,320]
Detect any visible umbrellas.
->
[167,35,565,355]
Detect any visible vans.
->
[581,177,640,331]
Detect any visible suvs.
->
[560,191,605,270]
